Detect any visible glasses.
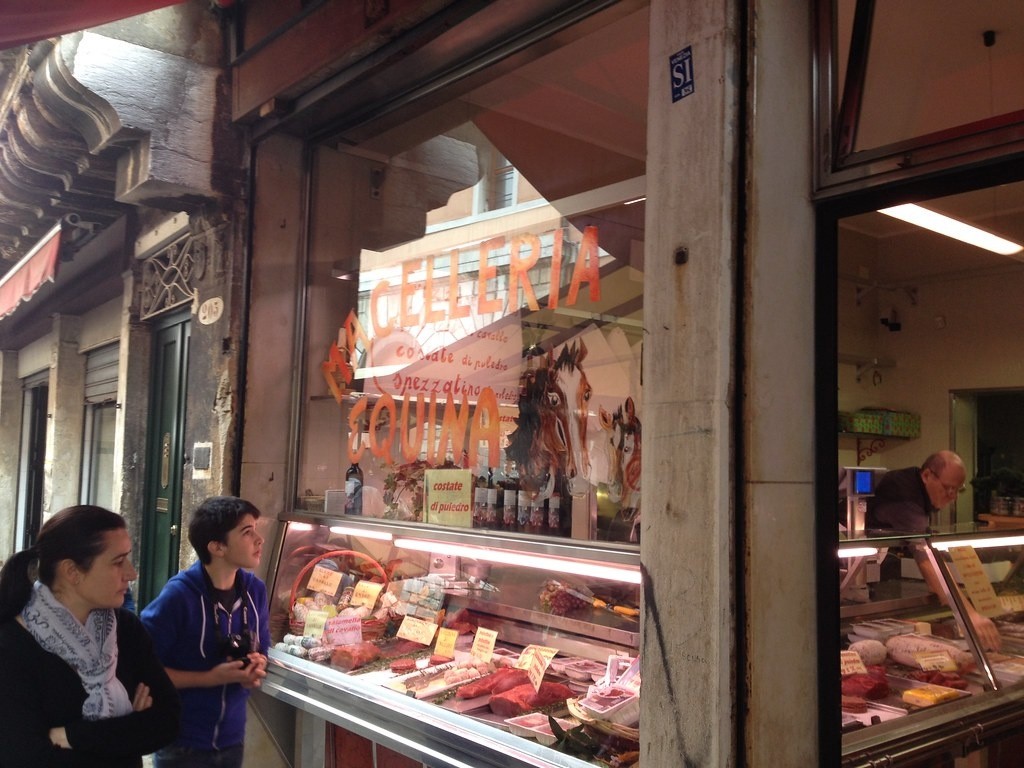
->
[929,469,968,495]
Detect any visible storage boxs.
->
[840,405,922,440]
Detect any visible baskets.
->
[288,549,388,644]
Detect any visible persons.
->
[0,505,176,768]
[866,450,1003,653]
[138,495,272,768]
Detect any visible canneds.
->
[336,587,354,611]
